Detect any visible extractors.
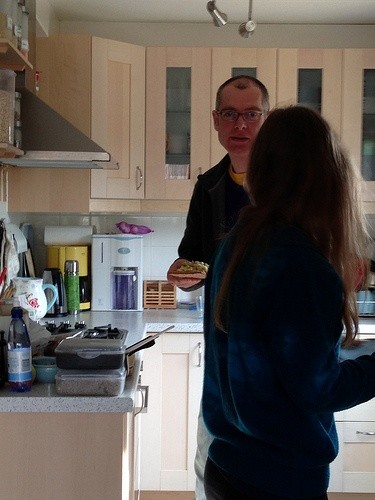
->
[20,88,120,170]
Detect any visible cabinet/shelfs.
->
[135,334,375,493]
[8,35,375,213]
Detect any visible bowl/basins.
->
[55,338,127,396]
[33,357,58,383]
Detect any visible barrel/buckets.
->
[0,69,16,145]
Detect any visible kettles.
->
[43,268,70,318]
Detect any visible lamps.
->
[207,0,228,27]
[238,0,256,38]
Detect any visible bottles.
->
[6,301,32,393]
[0,330,8,390]
[15,92,22,149]
[0,0,29,61]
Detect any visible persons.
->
[201,104,375,500]
[167,74,270,500]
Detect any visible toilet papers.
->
[44,225,96,246]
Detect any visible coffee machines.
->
[46,243,91,312]
[91,232,144,312]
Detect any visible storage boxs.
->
[55,337,126,395]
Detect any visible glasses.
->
[216,109,263,123]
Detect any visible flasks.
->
[64,260,80,315]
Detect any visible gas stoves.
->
[37,320,130,356]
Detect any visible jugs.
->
[12,276,58,324]
